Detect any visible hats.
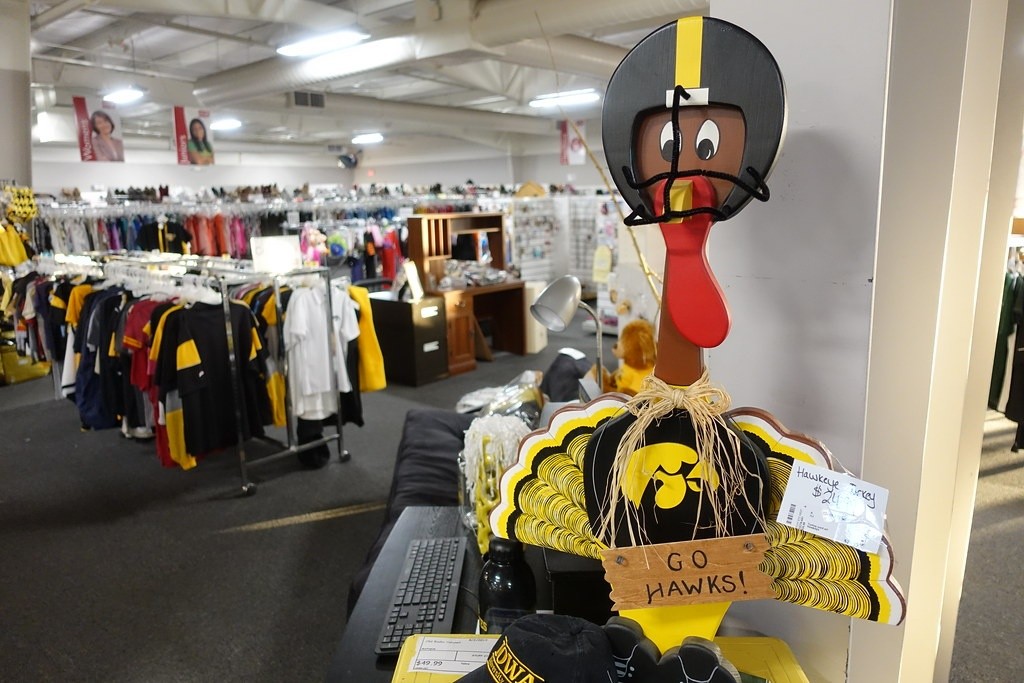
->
[452,610,620,683]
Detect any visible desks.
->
[325,505,487,683]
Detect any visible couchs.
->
[347,409,479,622]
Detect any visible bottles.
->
[479,536,538,636]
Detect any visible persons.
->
[186,119,215,164]
[90,110,123,161]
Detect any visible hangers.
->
[13,249,357,312]
[30,190,482,226]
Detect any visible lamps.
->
[531,274,605,403]
